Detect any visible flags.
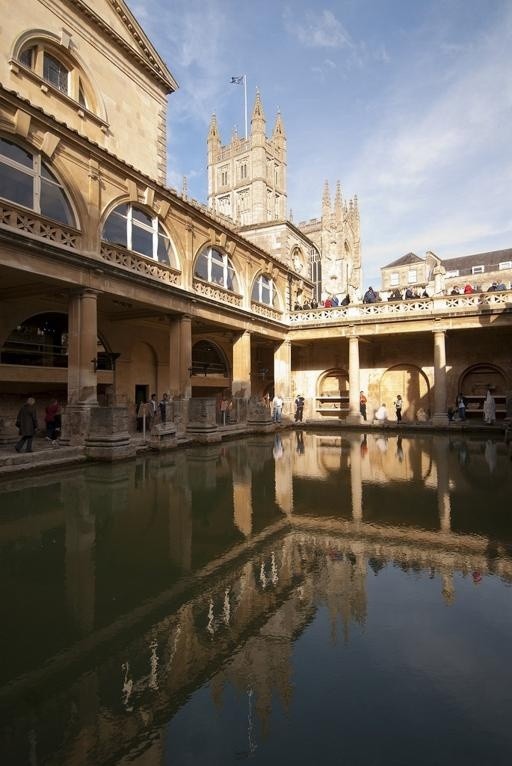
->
[230,76,245,87]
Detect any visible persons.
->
[147,394,159,432]
[294,429,304,458]
[293,395,305,423]
[360,434,368,458]
[44,403,62,444]
[457,390,467,420]
[375,403,388,421]
[377,435,389,454]
[403,283,430,299]
[363,287,382,313]
[397,437,405,464]
[394,394,402,425]
[13,398,38,454]
[450,278,507,295]
[265,392,285,423]
[272,432,284,461]
[294,293,350,319]
[360,389,368,422]
[159,392,169,424]
